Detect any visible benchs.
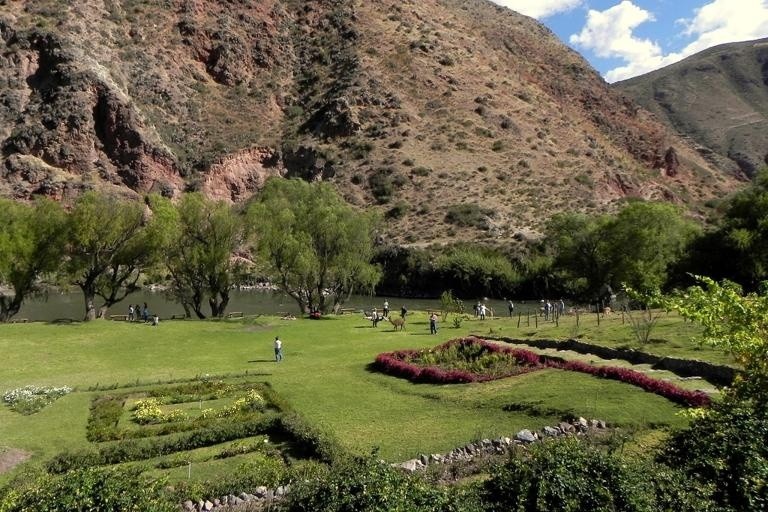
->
[173,313,186,318]
[426,309,443,315]
[372,308,391,315]
[141,313,156,322]
[230,311,244,318]
[341,307,357,314]
[110,314,130,321]
[12,319,29,323]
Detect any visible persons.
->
[382,298,389,317]
[400,304,407,321]
[479,303,489,320]
[282,309,321,321]
[371,307,378,328]
[151,315,159,325]
[475,301,481,317]
[141,302,150,323]
[134,304,141,321]
[539,298,565,321]
[128,304,134,321]
[507,300,514,318]
[273,336,282,362]
[429,312,438,334]
